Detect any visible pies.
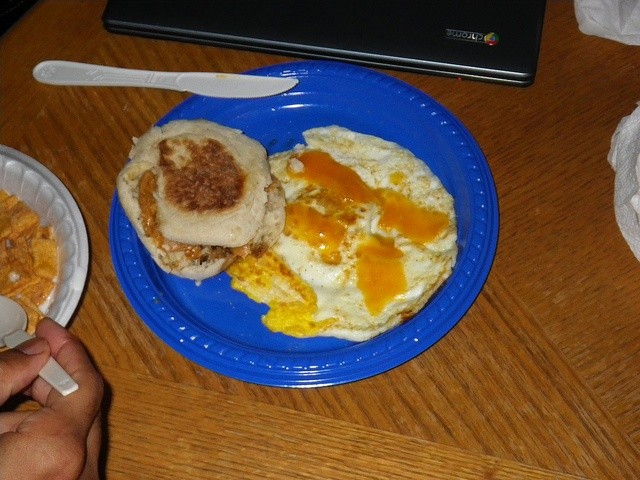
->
[1,191,56,347]
[117,118,285,277]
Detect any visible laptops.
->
[101,0,548,88]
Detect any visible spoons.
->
[0,295,78,397]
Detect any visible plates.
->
[109,62,500,392]
[0,143,91,354]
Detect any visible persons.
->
[0,317,106,480]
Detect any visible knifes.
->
[33,59,301,100]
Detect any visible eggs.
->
[226,124,459,344]
